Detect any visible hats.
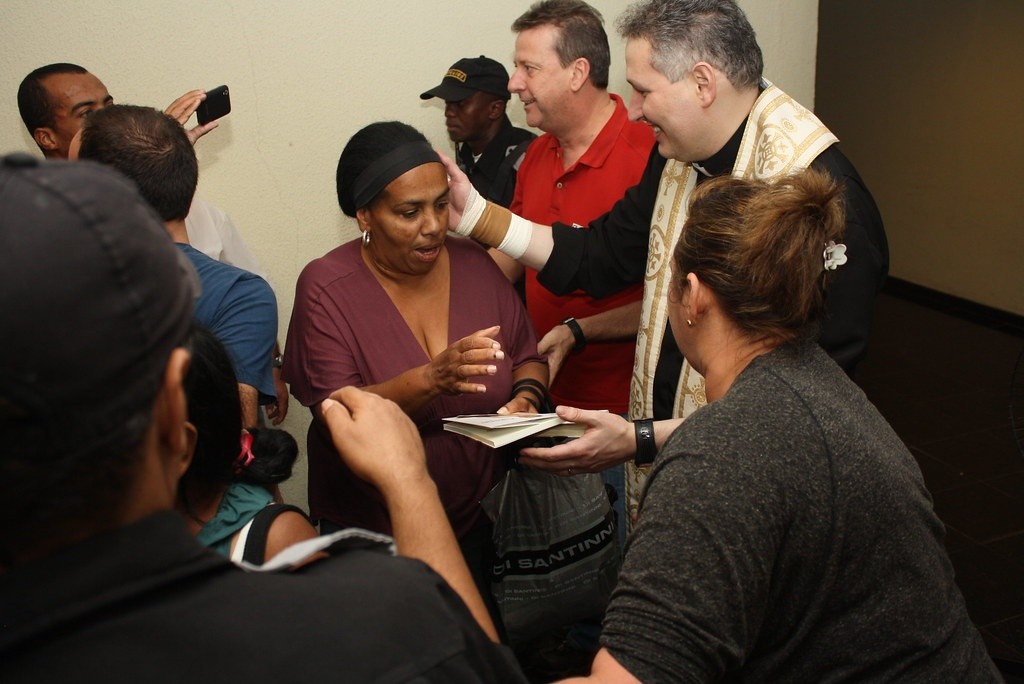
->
[0,153,201,458]
[338,121,444,217]
[421,55,518,104]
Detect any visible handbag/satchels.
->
[479,465,616,651]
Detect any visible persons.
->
[549,161,1005,684]
[421,53,538,249]
[283,121,547,573]
[0,63,326,568]
[0,147,527,684]
[436,1,891,480]
[484,1,655,547]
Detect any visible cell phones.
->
[195,86,232,126]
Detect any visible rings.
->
[569,468,571,472]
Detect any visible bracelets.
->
[273,354,283,366]
[560,317,587,348]
[633,418,658,467]
[454,182,533,260]
